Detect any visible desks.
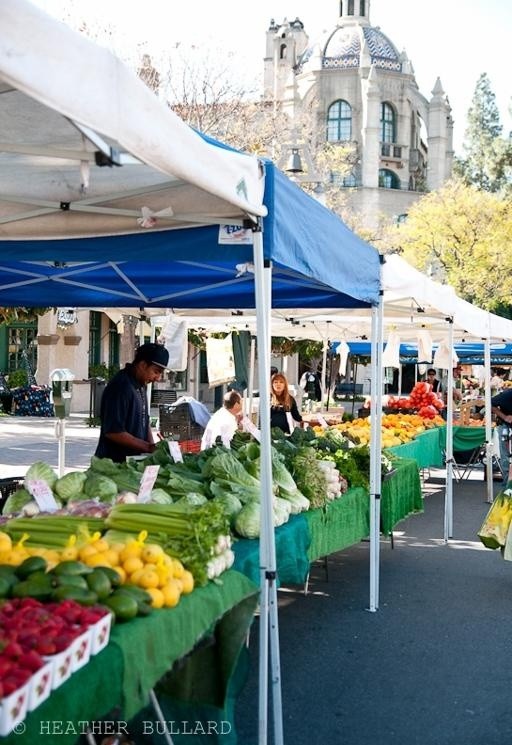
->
[381,424,494,550]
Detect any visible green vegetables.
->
[0,504,235,588]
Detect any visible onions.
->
[0,499,112,527]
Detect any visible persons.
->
[492,368,506,382]
[94,343,169,463]
[271,366,278,376]
[436,368,462,402]
[491,388,512,486]
[259,373,303,434]
[422,369,442,392]
[200,391,242,451]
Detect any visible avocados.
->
[0,556,152,627]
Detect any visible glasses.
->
[428,373,435,376]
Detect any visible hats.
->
[135,342,176,375]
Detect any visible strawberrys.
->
[0,596,110,699]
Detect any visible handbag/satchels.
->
[478,485,512,550]
[501,519,512,563]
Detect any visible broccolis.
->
[272,426,315,444]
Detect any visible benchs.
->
[334,382,364,400]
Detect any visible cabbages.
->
[1,444,310,539]
